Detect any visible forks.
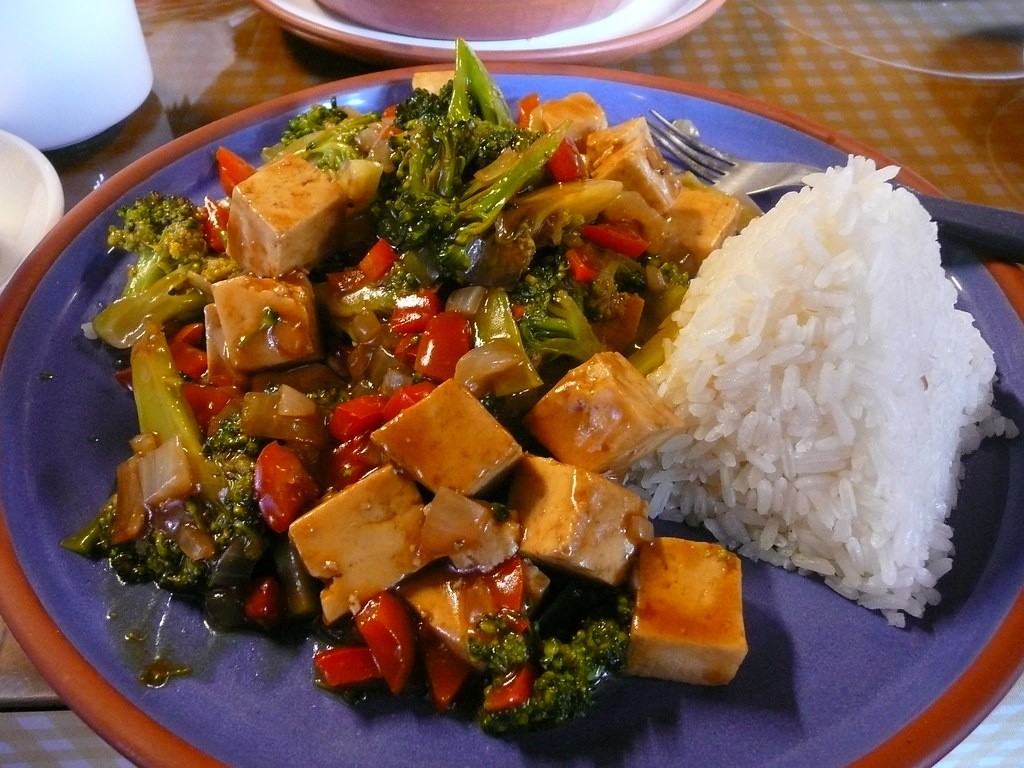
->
[646,108,1024,251]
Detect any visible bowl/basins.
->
[316,0,634,42]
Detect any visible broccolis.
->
[61,38,706,734]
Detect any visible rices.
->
[614,153,1019,627]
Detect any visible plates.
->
[0,64,1024,768]
[0,129,67,304]
[251,0,728,64]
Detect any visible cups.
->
[0,0,154,154]
